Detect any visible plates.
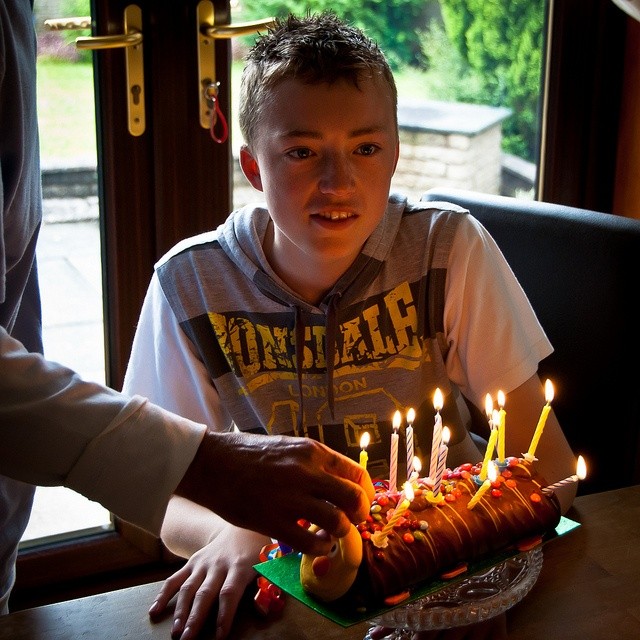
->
[368,545,544,631]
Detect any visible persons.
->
[0,0,376,619]
[119,5,582,640]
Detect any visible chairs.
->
[419,190,639,498]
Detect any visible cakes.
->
[299,456,561,604]
